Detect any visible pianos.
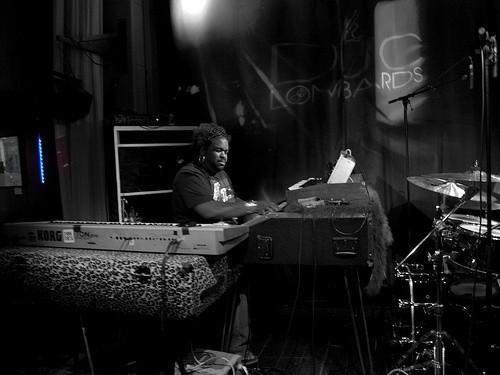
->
[0,220,249,254]
[230,202,375,269]
[286,178,366,203]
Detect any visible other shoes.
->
[245,350,258,365]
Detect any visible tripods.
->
[390,252,479,375]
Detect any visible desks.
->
[0,247,242,375]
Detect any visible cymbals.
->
[422,171,500,182]
[406,176,497,203]
[439,201,500,210]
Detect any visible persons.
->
[171,122,282,366]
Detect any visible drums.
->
[427,255,500,298]
[439,213,500,248]
[447,224,500,278]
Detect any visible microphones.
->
[468,56,474,89]
[459,187,479,202]
[493,36,498,77]
